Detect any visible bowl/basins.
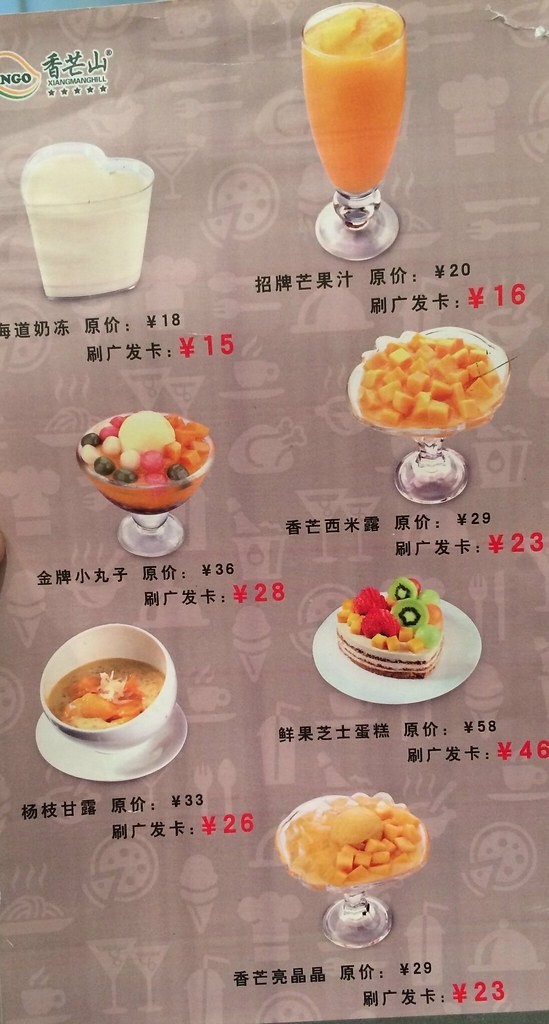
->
[39,623,178,754]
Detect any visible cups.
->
[18,140,155,299]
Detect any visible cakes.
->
[337,578,444,681]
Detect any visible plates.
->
[312,591,483,705]
[35,701,188,782]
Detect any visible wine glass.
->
[274,792,430,950]
[347,326,512,504]
[301,2,408,262]
[76,411,216,558]
